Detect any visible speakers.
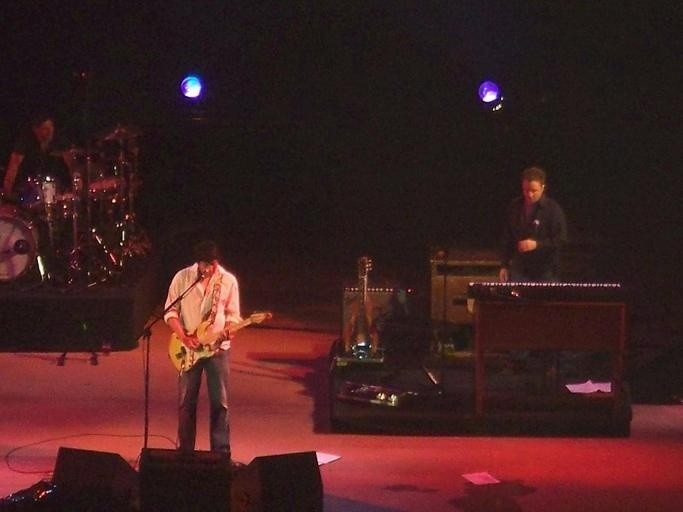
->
[138,448,231,512]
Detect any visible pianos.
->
[466,280,633,438]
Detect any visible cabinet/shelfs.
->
[429,258,504,324]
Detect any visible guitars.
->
[169,310,273,372]
[343,256,378,360]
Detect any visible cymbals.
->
[100,125,143,141]
[63,145,97,162]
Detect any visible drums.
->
[15,172,56,214]
[0,205,37,283]
[88,176,123,214]
[54,194,81,221]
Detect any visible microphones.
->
[195,268,210,284]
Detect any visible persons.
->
[163,238,241,455]
[0,112,71,211]
[499,165,567,282]
